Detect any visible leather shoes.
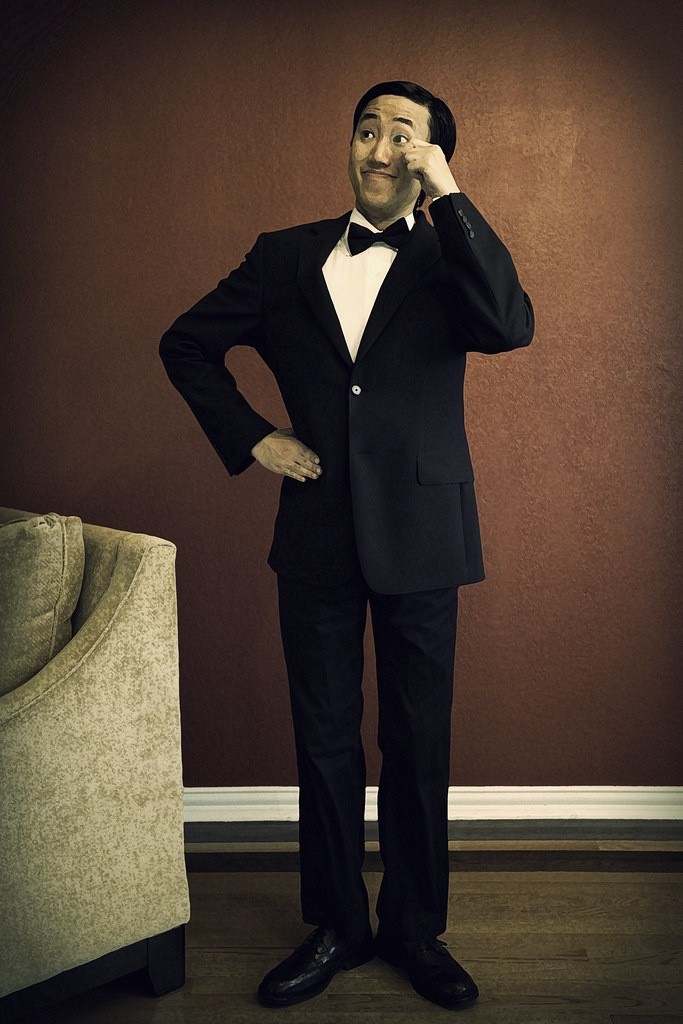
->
[373,919,478,1008]
[256,923,375,1007]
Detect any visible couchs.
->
[0,514,189,1005]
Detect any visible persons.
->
[158,80,534,1012]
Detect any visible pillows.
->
[2,512,82,691]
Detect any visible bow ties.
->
[347,217,408,256]
